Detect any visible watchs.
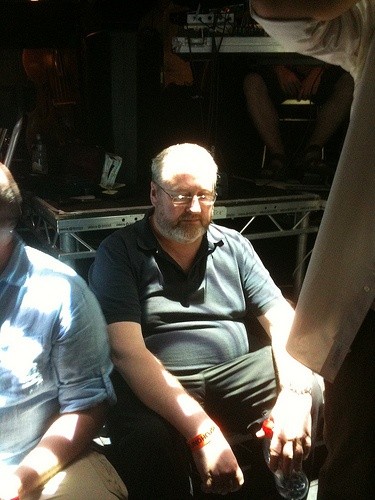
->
[280,376,314,397]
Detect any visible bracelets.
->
[186,425,218,451]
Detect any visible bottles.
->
[263,419,308,500]
[32,133,47,173]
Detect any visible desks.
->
[20,181,324,300]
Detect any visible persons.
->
[0,163,129,499]
[86,141,321,500]
[242,51,354,182]
[248,0,375,500]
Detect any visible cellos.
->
[24,48,106,158]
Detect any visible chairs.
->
[258,95,330,171]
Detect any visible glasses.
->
[151,178,219,206]
